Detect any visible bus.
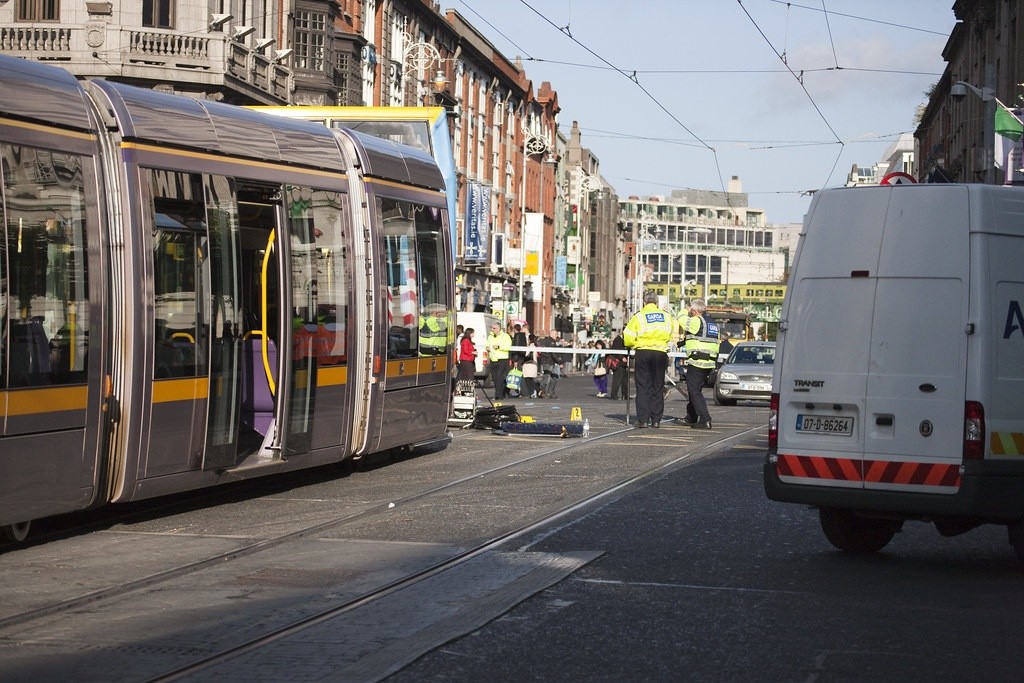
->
[0,55,517,548]
[705,308,756,388]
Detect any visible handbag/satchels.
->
[506,366,523,389]
[606,356,619,370]
[522,361,537,378]
[594,360,606,376]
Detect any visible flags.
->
[994,106,1024,170]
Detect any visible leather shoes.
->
[652,422,660,427]
[674,417,692,425]
[639,422,648,428]
[691,420,711,429]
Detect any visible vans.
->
[455,312,503,388]
[764,183,1024,554]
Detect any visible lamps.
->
[653,226,664,236]
[274,49,294,64]
[86,2,113,19]
[254,39,276,54]
[233,26,257,42]
[544,153,558,169]
[653,243,657,252]
[430,66,450,93]
[208,13,234,31]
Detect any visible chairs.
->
[1,323,282,436]
[742,351,773,364]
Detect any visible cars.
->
[712,341,776,407]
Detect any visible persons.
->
[487,323,561,401]
[590,340,608,397]
[454,325,481,402]
[610,328,634,400]
[555,336,612,371]
[677,301,719,429]
[624,292,674,427]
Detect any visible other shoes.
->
[621,394,632,400]
[599,393,607,397]
[519,388,559,400]
[611,397,622,401]
[597,391,602,397]
